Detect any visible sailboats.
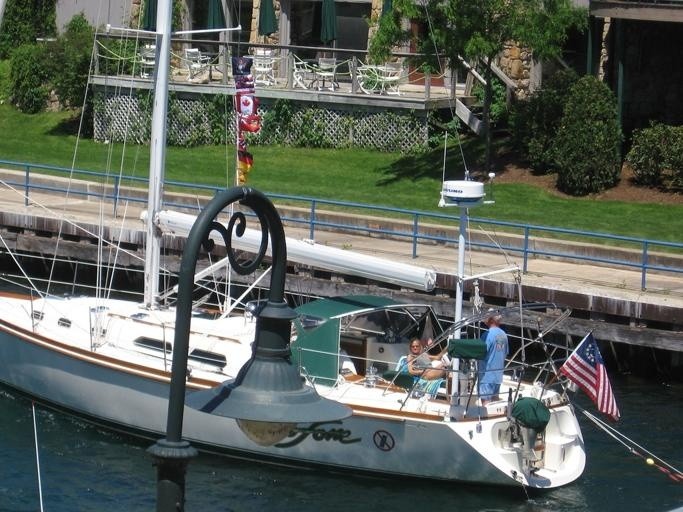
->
[0,1,588,493]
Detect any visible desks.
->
[243,55,282,86]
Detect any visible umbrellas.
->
[143,0,403,59]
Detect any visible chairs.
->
[140,44,157,79]
[184,48,212,82]
[396,355,447,399]
[251,49,406,97]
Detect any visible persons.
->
[394,337,450,382]
[477,307,509,406]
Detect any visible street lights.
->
[146,185,353,512]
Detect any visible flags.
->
[232,56,263,188]
[559,331,621,423]
